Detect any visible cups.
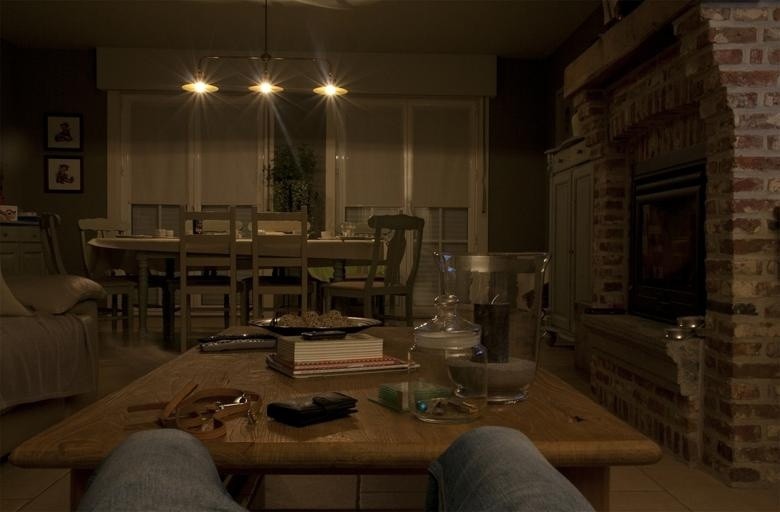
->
[152,229,165,237]
[341,222,356,236]
[434,251,553,405]
[165,231,173,237]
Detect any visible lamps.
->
[311,74,350,98]
[249,65,285,97]
[180,70,221,97]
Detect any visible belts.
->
[126,381,264,441]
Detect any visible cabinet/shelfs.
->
[546,137,595,347]
[0,218,50,277]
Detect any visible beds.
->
[1,275,106,409]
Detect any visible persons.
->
[78,426,596,511]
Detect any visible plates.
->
[249,316,382,338]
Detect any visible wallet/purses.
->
[266,389,360,428]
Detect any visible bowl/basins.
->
[321,231,332,238]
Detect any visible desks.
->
[9,322,662,512]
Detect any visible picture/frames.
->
[45,155,85,194]
[38,109,87,152]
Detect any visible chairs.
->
[78,217,173,341]
[322,213,425,329]
[173,211,239,352]
[77,217,139,344]
[40,212,138,335]
[247,203,311,318]
